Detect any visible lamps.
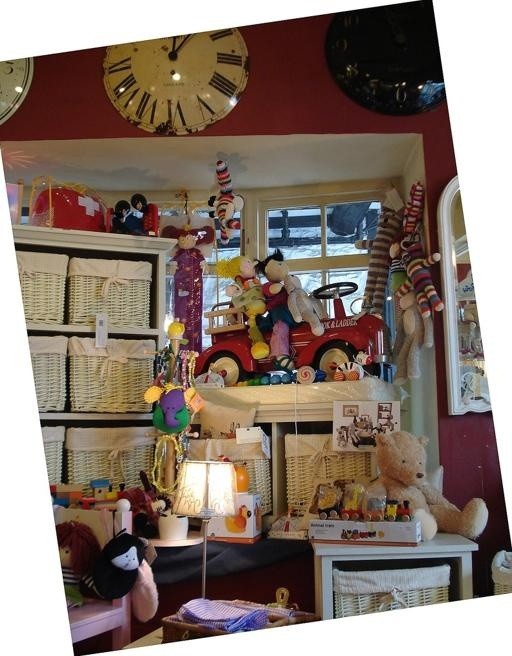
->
[171,459,240,600]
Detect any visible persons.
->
[365,427,489,541]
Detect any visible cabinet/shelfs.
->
[11,224,179,492]
[189,375,480,620]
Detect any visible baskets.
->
[162,600,322,644]
[187,434,371,515]
[15,251,157,491]
[491,550,512,595]
[333,564,450,618]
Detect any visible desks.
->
[151,530,205,547]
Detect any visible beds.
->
[51,496,132,651]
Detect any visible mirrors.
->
[437,174,492,416]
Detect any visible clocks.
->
[102,27,250,136]
[323,0,446,117]
[0,57,35,126]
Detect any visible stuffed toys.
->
[172,158,442,386]
[111,198,130,232]
[55,520,100,603]
[130,192,159,236]
[79,531,163,625]
